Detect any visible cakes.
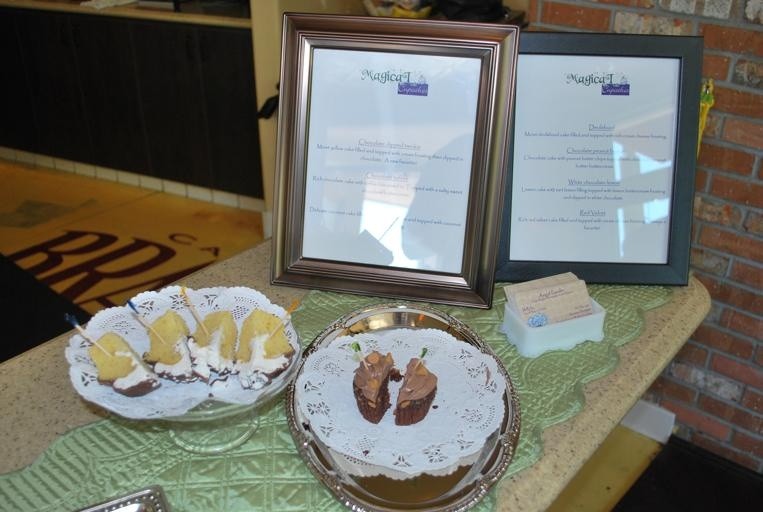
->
[142,309,196,382]
[87,331,161,398]
[352,352,394,423]
[395,357,436,425]
[235,309,294,391]
[187,312,238,383]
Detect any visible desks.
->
[0,239,711,511]
